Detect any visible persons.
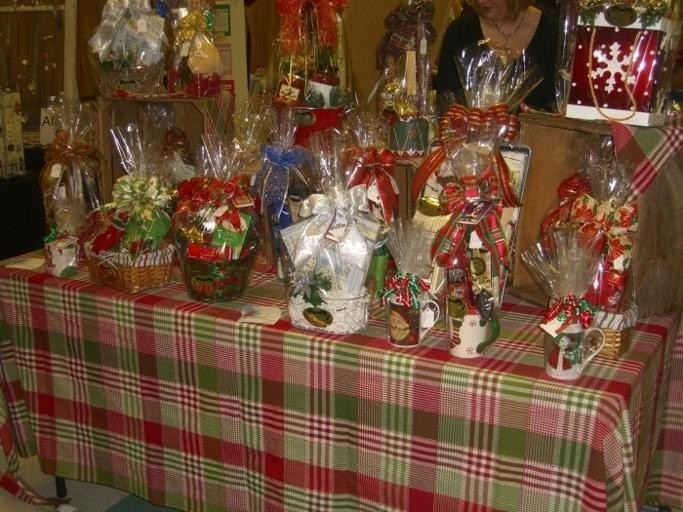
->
[434,0,565,115]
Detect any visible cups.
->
[386,299,440,349]
[445,301,501,359]
[544,322,605,382]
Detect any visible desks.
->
[0,249,677,512]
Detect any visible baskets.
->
[84,242,174,296]
[287,292,371,334]
